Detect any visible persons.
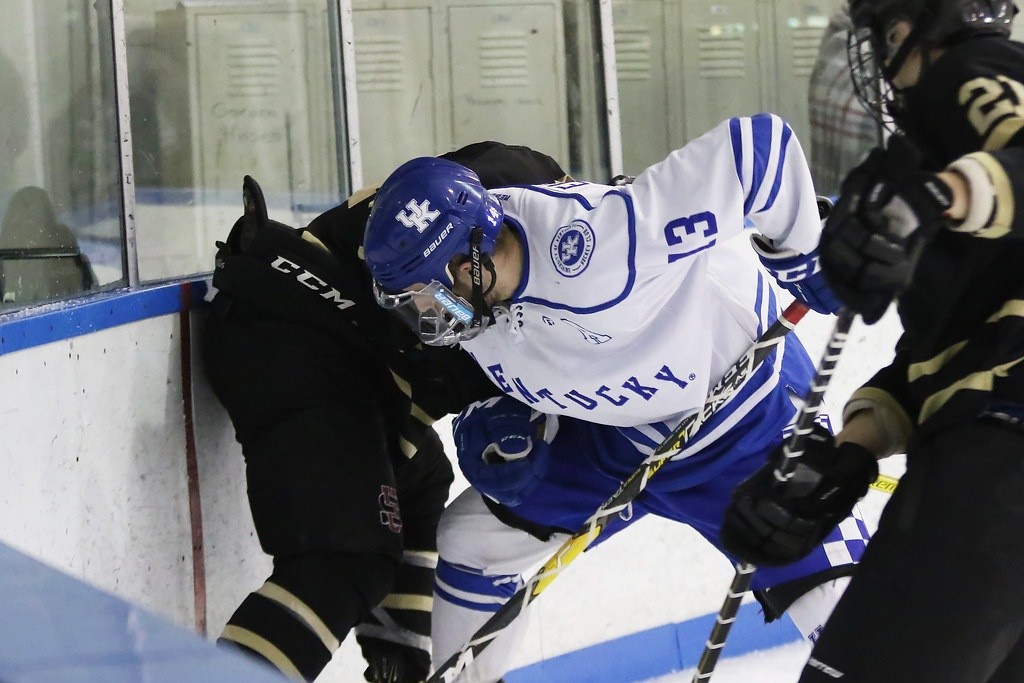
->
[198,2,1024,683]
[362,113,872,683]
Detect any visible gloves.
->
[821,144,954,325]
[721,423,879,567]
[453,396,562,507]
[749,196,841,317]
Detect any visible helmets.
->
[364,157,503,346]
[846,0,1021,137]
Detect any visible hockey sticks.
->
[691,305,858,682]
[422,295,812,682]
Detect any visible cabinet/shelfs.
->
[156,1,573,277]
[573,1,849,183]
